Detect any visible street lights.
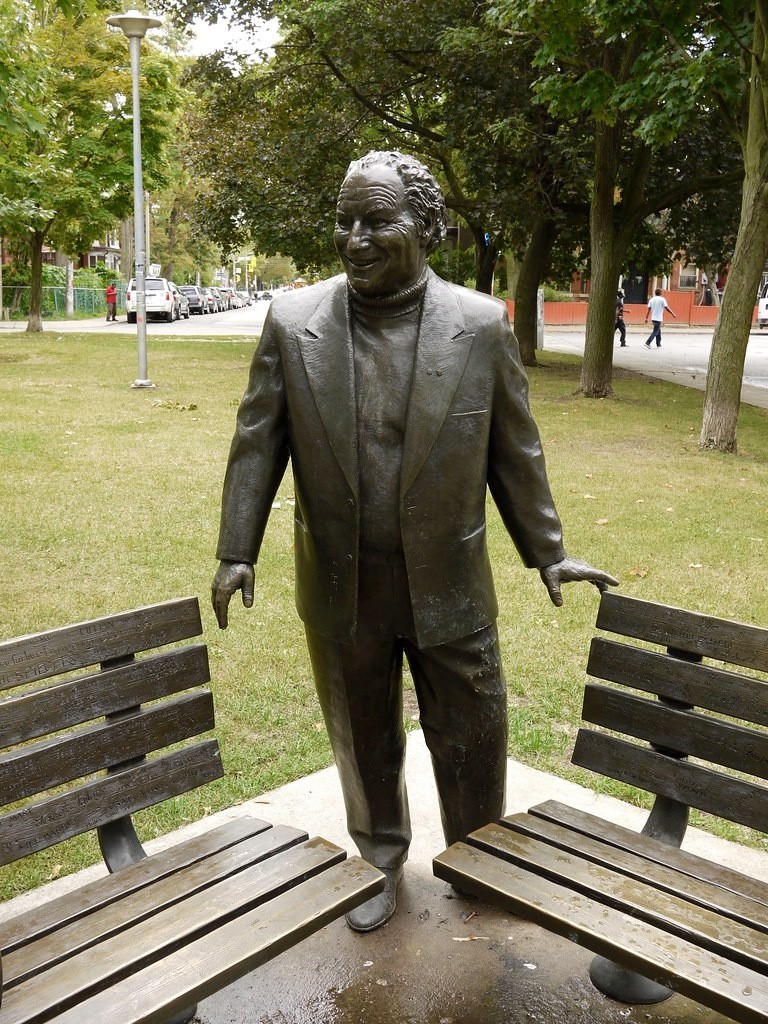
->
[105,10,162,390]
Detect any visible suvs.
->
[125,276,209,323]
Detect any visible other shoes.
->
[645,342,651,349]
[621,344,629,347]
[112,318,118,321]
[107,317,111,321]
[657,344,662,347]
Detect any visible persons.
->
[211,150,620,934]
[106,284,119,321]
[615,291,631,347]
[645,288,676,349]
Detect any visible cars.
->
[205,288,252,314]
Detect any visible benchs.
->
[433,592,768,1022]
[0,596,388,1024]
